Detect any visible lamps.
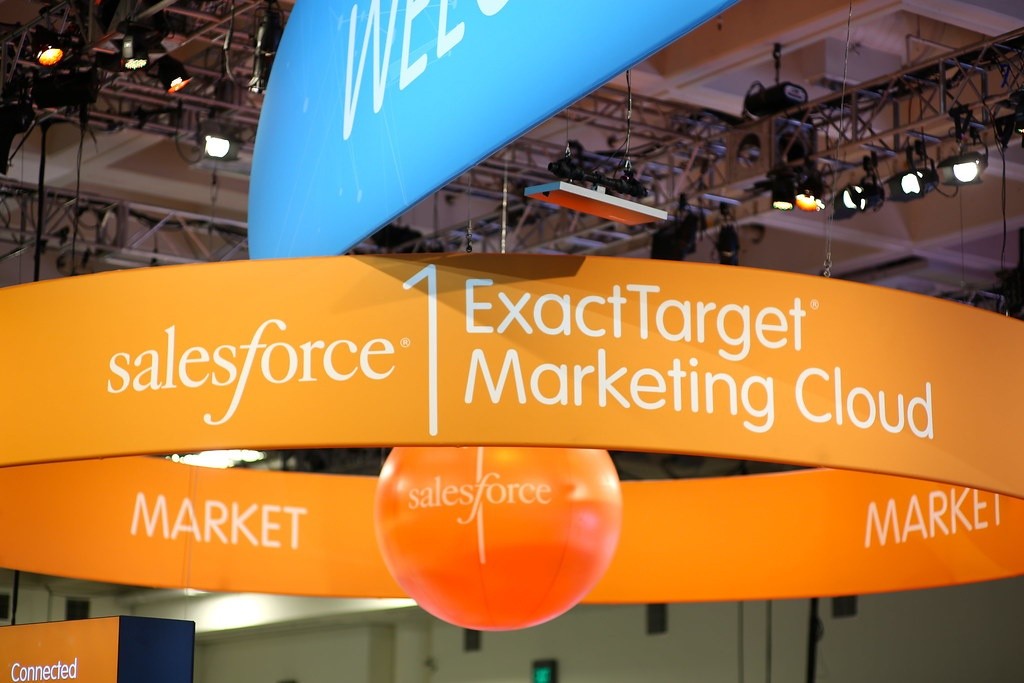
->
[841,184,885,211]
[900,168,939,196]
[936,151,987,185]
[796,174,825,212]
[767,159,795,212]
[31,23,65,67]
[156,54,194,95]
[370,183,623,630]
[204,134,246,161]
[122,33,150,72]
